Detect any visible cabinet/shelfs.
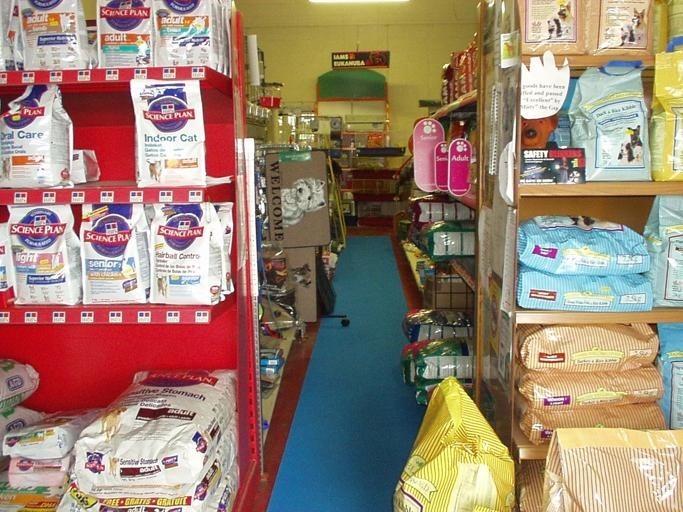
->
[332,146,405,238]
